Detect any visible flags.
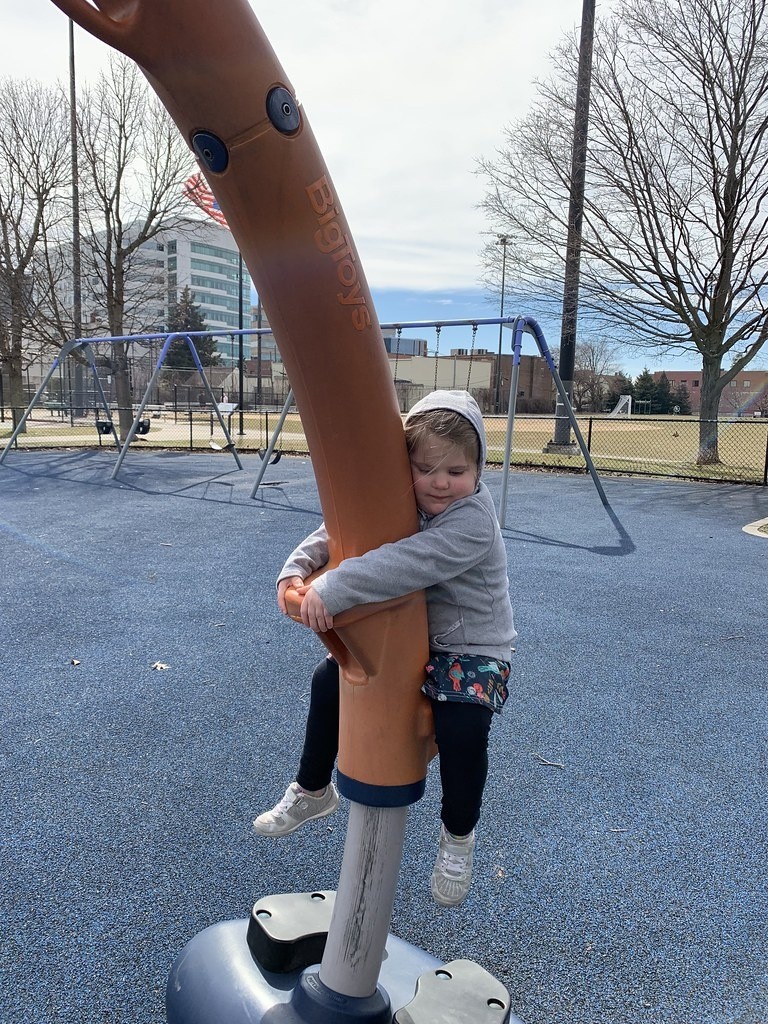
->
[184,174,231,233]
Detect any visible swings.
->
[207,336,236,450]
[94,338,113,435]
[131,339,152,434]
[256,333,284,465]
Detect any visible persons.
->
[252,390,518,907]
[218,393,229,412]
[198,391,209,416]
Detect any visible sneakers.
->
[431,823,476,907]
[253,780,339,837]
